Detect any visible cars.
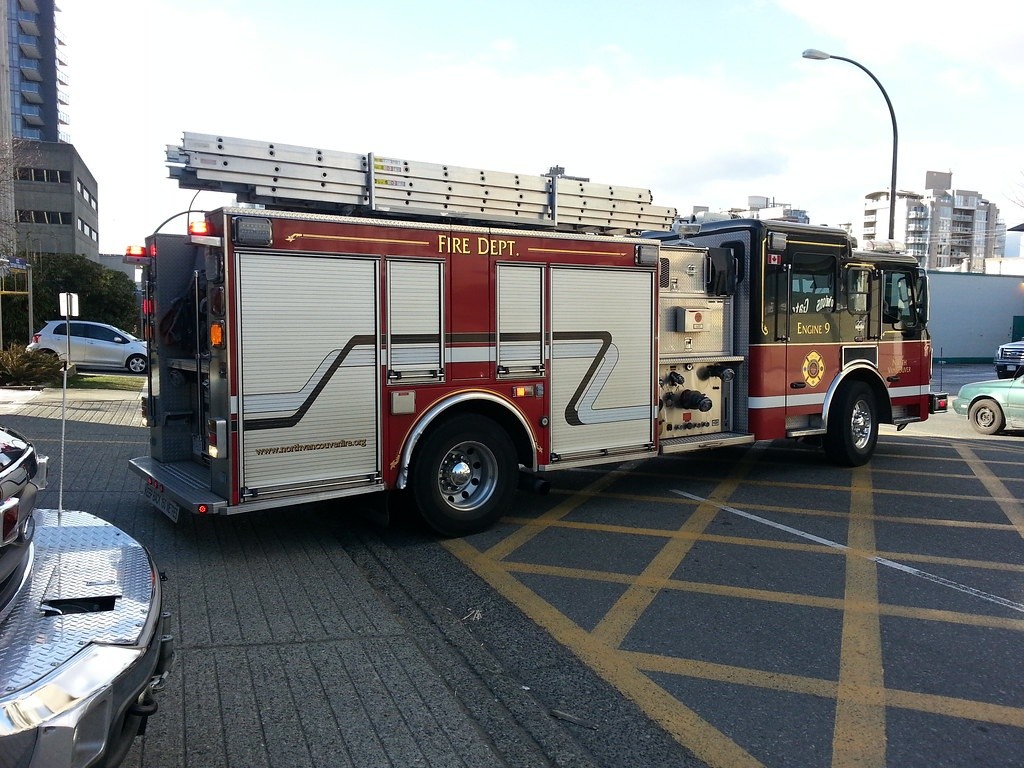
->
[951,372,1024,435]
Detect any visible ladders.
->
[165,131,676,235]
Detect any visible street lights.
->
[801,48,899,241]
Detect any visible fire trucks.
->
[123,132,950,537]
[1,359,176,768]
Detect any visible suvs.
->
[25,321,149,375]
[992,336,1024,381]
